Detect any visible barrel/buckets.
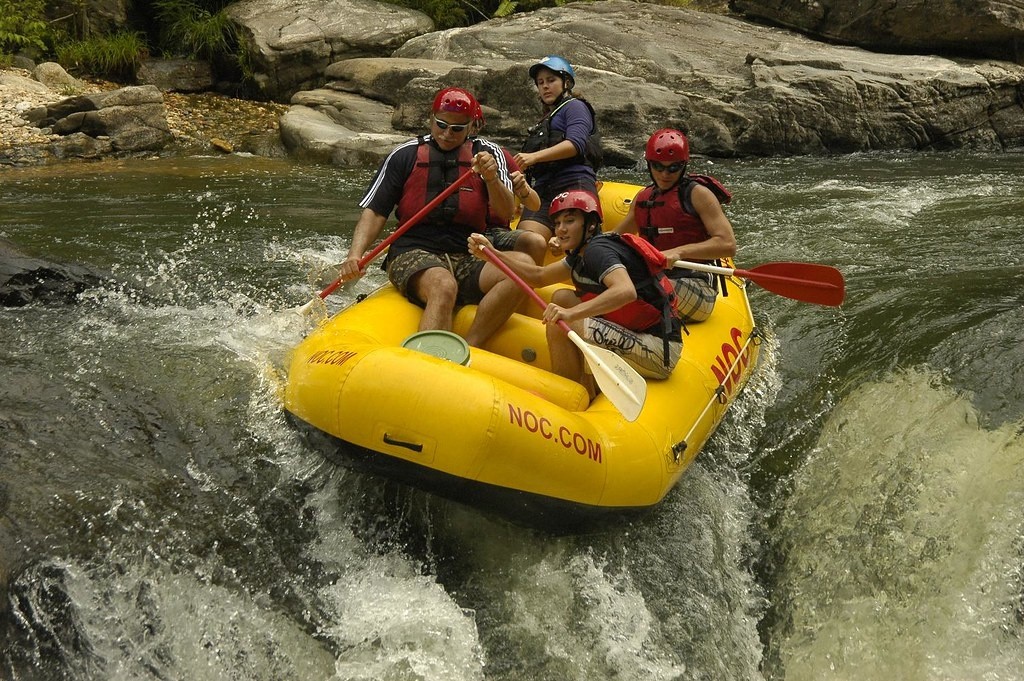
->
[402,330,471,368]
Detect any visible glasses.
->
[650,160,684,173]
[433,114,473,132]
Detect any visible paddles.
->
[673,259,844,306]
[294,154,479,316]
[322,244,390,290]
[477,242,648,422]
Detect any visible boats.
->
[283,182,762,538]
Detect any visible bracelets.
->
[521,190,530,198]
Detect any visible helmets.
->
[548,190,603,224]
[645,129,689,161]
[529,55,575,90]
[432,88,477,120]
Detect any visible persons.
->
[468,101,547,267]
[341,88,536,349]
[613,129,737,321]
[467,189,683,401]
[513,55,604,242]
